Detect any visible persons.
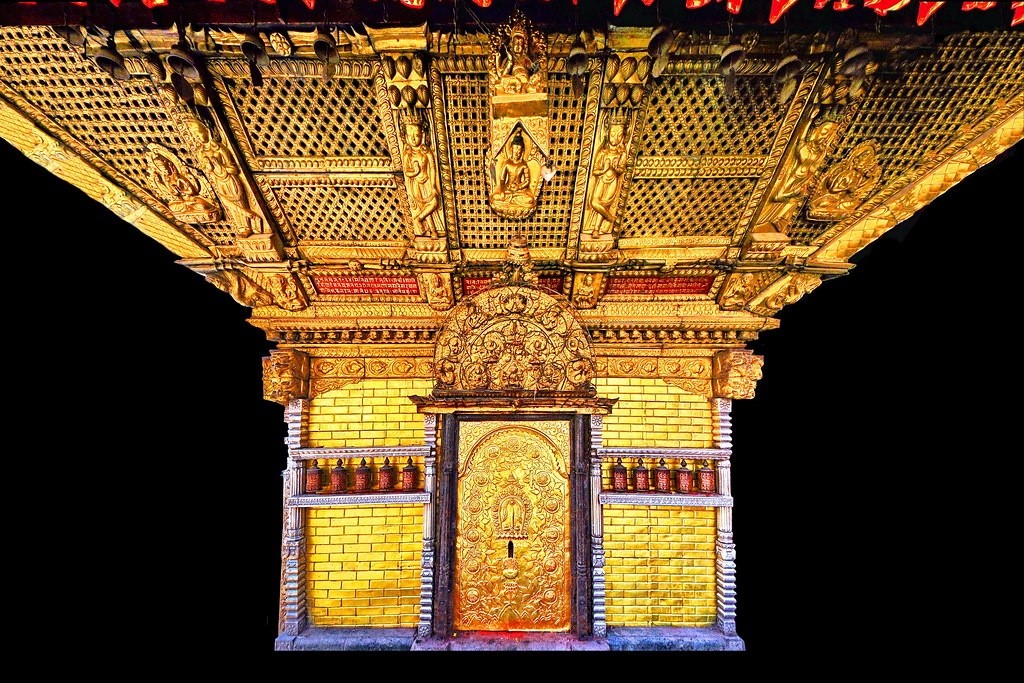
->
[401,108,444,240]
[491,135,536,217]
[430,274,450,305]
[576,273,595,306]
[273,274,303,311]
[151,152,211,212]
[766,108,840,233]
[725,273,752,307]
[588,108,626,238]
[496,24,539,94]
[814,147,874,215]
[176,97,263,239]
